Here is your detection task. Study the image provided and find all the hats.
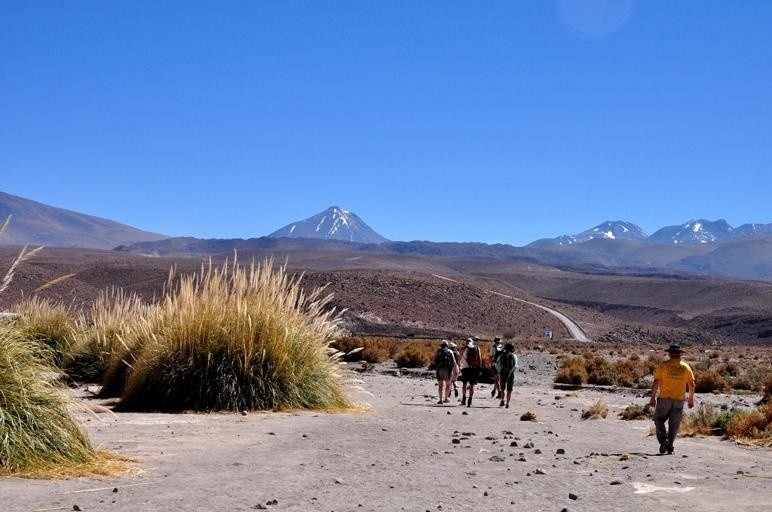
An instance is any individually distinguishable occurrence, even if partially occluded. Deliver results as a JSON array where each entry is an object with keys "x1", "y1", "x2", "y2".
[
  {"x1": 664, "y1": 343, "x2": 686, "y2": 355},
  {"x1": 440, "y1": 335, "x2": 501, "y2": 347}
]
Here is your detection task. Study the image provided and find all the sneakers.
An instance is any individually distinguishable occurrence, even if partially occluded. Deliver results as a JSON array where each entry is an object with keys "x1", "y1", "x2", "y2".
[
  {"x1": 659, "y1": 440, "x2": 675, "y2": 454},
  {"x1": 437, "y1": 388, "x2": 510, "y2": 409}
]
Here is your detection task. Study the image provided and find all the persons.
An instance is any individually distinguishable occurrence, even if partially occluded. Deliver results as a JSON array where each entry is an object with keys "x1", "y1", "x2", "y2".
[
  {"x1": 648, "y1": 344, "x2": 695, "y2": 453},
  {"x1": 495, "y1": 343, "x2": 518, "y2": 408},
  {"x1": 489, "y1": 337, "x2": 502, "y2": 398},
  {"x1": 457, "y1": 338, "x2": 482, "y2": 407},
  {"x1": 430, "y1": 340, "x2": 456, "y2": 404},
  {"x1": 448, "y1": 342, "x2": 460, "y2": 397}
]
